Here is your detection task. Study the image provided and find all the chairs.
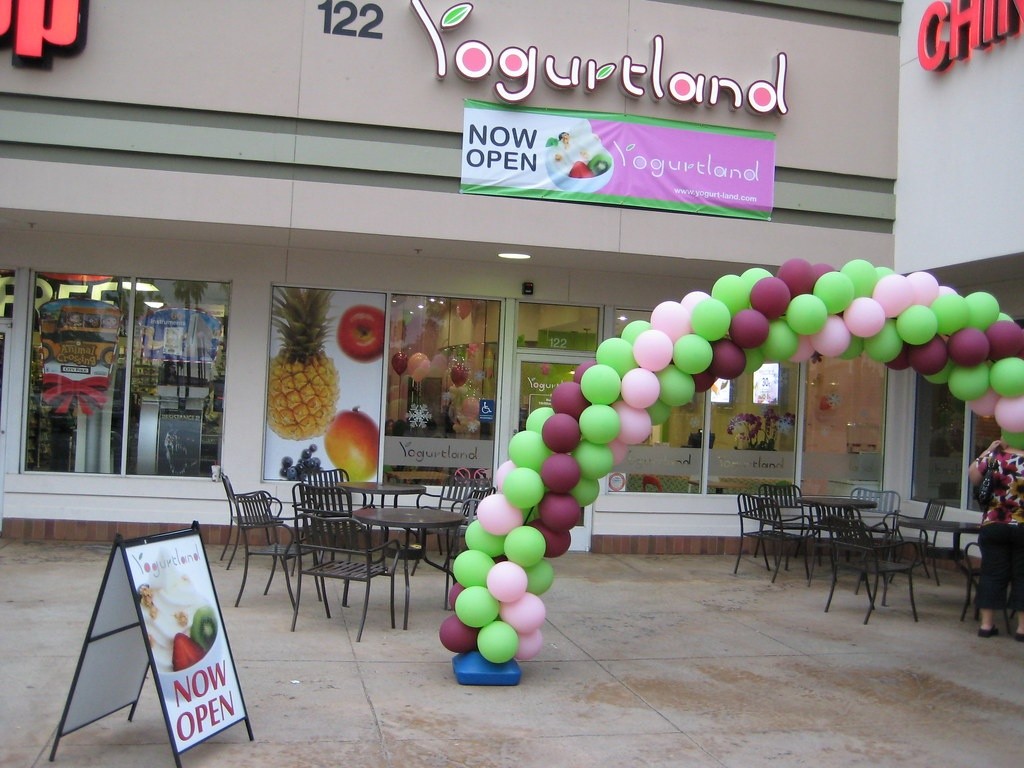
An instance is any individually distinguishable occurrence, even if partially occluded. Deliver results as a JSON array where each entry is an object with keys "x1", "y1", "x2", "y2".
[
  {"x1": 734, "y1": 484, "x2": 1015, "y2": 637},
  {"x1": 221, "y1": 468, "x2": 496, "y2": 641}
]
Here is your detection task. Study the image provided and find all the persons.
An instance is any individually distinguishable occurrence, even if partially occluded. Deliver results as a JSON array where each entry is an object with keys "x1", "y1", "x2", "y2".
[
  {"x1": 967, "y1": 435, "x2": 1024, "y2": 642},
  {"x1": 386, "y1": 417, "x2": 437, "y2": 435},
  {"x1": 519, "y1": 415, "x2": 528, "y2": 431}
]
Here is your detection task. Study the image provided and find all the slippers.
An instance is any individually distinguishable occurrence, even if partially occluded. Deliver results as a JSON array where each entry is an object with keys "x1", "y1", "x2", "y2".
[
  {"x1": 978, "y1": 624, "x2": 999, "y2": 637},
  {"x1": 1015, "y1": 633, "x2": 1024, "y2": 641}
]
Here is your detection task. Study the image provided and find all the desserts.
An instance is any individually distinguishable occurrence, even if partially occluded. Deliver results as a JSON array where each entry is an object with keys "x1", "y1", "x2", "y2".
[
  {"x1": 139, "y1": 546, "x2": 218, "y2": 673},
  {"x1": 548, "y1": 119, "x2": 601, "y2": 175}
]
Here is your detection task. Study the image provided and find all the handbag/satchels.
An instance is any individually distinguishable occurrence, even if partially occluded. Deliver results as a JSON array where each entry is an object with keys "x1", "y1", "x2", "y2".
[{"x1": 978, "y1": 447, "x2": 1000, "y2": 504}]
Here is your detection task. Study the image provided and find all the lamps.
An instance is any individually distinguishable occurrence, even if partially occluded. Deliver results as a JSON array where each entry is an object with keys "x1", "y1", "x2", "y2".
[{"x1": 568, "y1": 331, "x2": 577, "y2": 375}]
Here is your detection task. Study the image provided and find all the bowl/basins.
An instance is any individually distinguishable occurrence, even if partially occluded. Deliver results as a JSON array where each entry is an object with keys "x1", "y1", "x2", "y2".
[
  {"x1": 157, "y1": 625, "x2": 221, "y2": 701},
  {"x1": 546, "y1": 146, "x2": 613, "y2": 193}
]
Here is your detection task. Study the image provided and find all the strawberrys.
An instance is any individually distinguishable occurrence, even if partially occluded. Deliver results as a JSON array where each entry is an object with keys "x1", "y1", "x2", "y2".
[
  {"x1": 171, "y1": 633, "x2": 205, "y2": 672},
  {"x1": 568, "y1": 161, "x2": 595, "y2": 178}
]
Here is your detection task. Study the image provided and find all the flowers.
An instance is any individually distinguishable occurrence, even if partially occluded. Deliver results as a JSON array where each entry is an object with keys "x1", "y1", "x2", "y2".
[{"x1": 727, "y1": 407, "x2": 795, "y2": 450}]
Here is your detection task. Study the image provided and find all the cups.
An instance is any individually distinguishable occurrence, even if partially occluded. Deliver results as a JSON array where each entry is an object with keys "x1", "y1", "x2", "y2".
[{"x1": 211, "y1": 465, "x2": 221, "y2": 483}]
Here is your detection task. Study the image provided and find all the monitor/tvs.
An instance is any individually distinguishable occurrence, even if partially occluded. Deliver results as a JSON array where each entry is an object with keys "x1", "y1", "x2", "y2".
[
  {"x1": 710, "y1": 378, "x2": 732, "y2": 406},
  {"x1": 752, "y1": 362, "x2": 782, "y2": 407}
]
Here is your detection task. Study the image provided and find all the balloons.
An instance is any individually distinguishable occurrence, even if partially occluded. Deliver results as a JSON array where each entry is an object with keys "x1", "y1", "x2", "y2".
[
  {"x1": 819, "y1": 395, "x2": 831, "y2": 411},
  {"x1": 391, "y1": 352, "x2": 431, "y2": 382},
  {"x1": 450, "y1": 365, "x2": 468, "y2": 387},
  {"x1": 439, "y1": 257, "x2": 1024, "y2": 663},
  {"x1": 463, "y1": 397, "x2": 479, "y2": 420},
  {"x1": 457, "y1": 300, "x2": 472, "y2": 320}
]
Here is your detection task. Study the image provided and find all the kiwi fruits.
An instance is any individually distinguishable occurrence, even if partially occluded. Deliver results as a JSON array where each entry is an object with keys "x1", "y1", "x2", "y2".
[
  {"x1": 587, "y1": 153, "x2": 612, "y2": 176},
  {"x1": 189, "y1": 606, "x2": 217, "y2": 652}
]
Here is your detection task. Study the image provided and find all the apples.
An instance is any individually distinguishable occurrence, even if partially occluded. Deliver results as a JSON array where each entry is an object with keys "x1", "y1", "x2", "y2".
[
  {"x1": 337, "y1": 304, "x2": 384, "y2": 363},
  {"x1": 324, "y1": 407, "x2": 378, "y2": 482}
]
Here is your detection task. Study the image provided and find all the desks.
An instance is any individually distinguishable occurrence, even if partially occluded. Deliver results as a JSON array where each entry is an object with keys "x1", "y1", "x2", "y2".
[
  {"x1": 896, "y1": 518, "x2": 981, "y2": 621},
  {"x1": 795, "y1": 496, "x2": 878, "y2": 572},
  {"x1": 351, "y1": 508, "x2": 465, "y2": 630},
  {"x1": 336, "y1": 481, "x2": 426, "y2": 575},
  {"x1": 383, "y1": 468, "x2": 450, "y2": 485},
  {"x1": 689, "y1": 479, "x2": 747, "y2": 494}
]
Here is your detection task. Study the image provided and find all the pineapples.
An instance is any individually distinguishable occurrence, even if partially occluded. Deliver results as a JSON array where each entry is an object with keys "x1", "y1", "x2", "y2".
[{"x1": 267, "y1": 285, "x2": 342, "y2": 441}]
